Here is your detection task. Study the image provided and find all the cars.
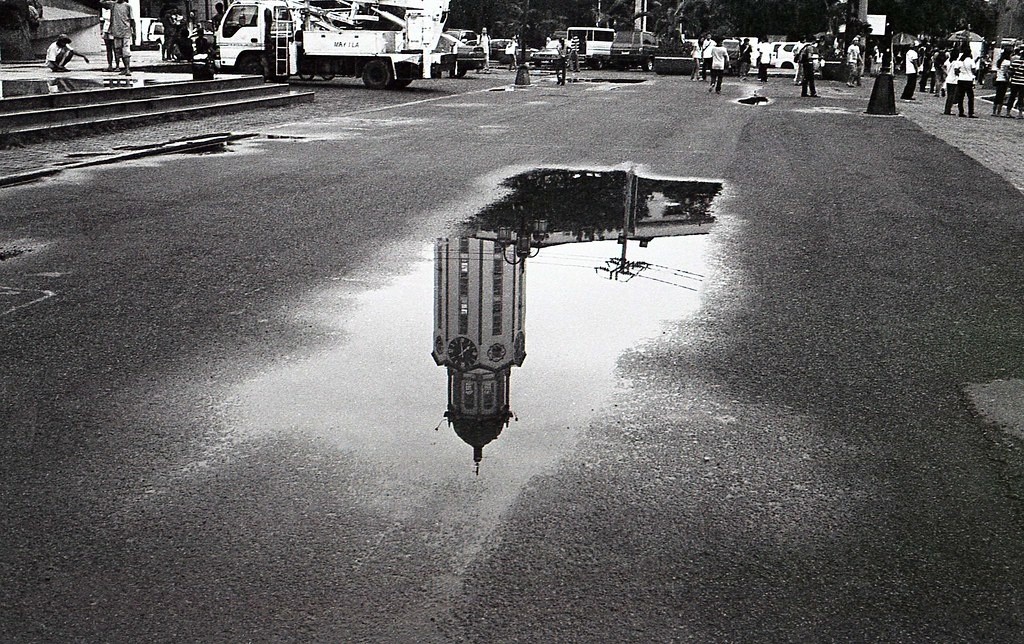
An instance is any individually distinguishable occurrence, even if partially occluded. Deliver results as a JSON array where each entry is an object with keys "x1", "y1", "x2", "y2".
[{"x1": 431, "y1": 27, "x2": 569, "y2": 78}]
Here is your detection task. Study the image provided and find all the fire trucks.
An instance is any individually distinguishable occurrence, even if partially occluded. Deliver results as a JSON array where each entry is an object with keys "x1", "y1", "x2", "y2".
[{"x1": 214, "y1": 0, "x2": 450, "y2": 91}]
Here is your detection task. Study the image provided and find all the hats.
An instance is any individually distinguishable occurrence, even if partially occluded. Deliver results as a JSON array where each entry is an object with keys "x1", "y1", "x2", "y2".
[{"x1": 57, "y1": 34, "x2": 72, "y2": 42}]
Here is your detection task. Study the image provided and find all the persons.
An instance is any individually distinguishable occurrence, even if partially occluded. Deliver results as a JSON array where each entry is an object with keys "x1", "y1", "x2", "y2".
[
  {"x1": 870, "y1": 40, "x2": 991, "y2": 118},
  {"x1": 991, "y1": 48, "x2": 1024, "y2": 119},
  {"x1": 555, "y1": 30, "x2": 580, "y2": 85},
  {"x1": 740, "y1": 36, "x2": 772, "y2": 82},
  {"x1": 505, "y1": 34, "x2": 518, "y2": 72},
  {"x1": 477, "y1": 28, "x2": 490, "y2": 74},
  {"x1": 29, "y1": 4, "x2": 40, "y2": 31},
  {"x1": 791, "y1": 35, "x2": 844, "y2": 97},
  {"x1": 690, "y1": 33, "x2": 730, "y2": 94},
  {"x1": 159, "y1": 0, "x2": 225, "y2": 60},
  {"x1": 546, "y1": 34, "x2": 552, "y2": 45},
  {"x1": 46, "y1": 34, "x2": 89, "y2": 71},
  {"x1": 846, "y1": 37, "x2": 866, "y2": 87},
  {"x1": 98, "y1": 0, "x2": 135, "y2": 76}
]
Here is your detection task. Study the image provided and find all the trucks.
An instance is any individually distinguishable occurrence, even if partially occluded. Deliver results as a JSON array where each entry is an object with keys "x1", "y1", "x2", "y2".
[
  {"x1": 139, "y1": 18, "x2": 166, "y2": 50},
  {"x1": 566, "y1": 26, "x2": 616, "y2": 70}
]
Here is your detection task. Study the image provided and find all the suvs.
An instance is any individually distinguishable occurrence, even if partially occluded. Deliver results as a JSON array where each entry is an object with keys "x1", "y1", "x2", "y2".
[
  {"x1": 689, "y1": 36, "x2": 800, "y2": 71},
  {"x1": 611, "y1": 31, "x2": 659, "y2": 73}
]
